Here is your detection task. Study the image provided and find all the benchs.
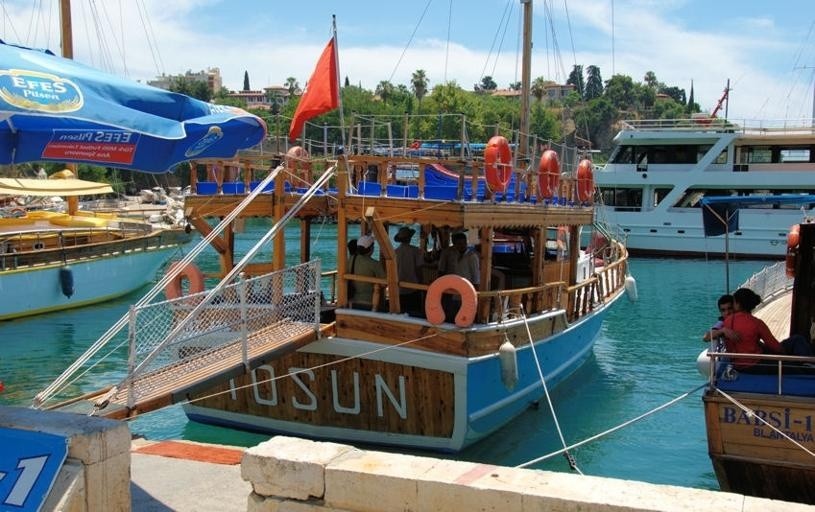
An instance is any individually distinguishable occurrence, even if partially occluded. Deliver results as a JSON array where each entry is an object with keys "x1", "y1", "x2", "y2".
[{"x1": 714, "y1": 352, "x2": 815, "y2": 397}]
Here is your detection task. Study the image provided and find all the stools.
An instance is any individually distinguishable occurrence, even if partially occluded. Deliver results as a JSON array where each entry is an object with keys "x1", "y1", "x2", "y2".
[{"x1": 494, "y1": 266, "x2": 513, "y2": 288}]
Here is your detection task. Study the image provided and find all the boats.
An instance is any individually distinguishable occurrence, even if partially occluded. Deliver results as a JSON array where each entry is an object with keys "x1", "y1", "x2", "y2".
[
  {"x1": 548, "y1": 107, "x2": 814, "y2": 262},
  {"x1": 695, "y1": 187, "x2": 815, "y2": 500}
]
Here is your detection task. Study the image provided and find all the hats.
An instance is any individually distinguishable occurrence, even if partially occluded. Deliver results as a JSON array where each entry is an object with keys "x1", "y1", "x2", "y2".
[
  {"x1": 357, "y1": 235, "x2": 374, "y2": 247},
  {"x1": 393, "y1": 226, "x2": 416, "y2": 242}
]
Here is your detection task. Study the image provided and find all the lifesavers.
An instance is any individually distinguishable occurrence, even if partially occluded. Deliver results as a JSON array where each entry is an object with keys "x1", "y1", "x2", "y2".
[
  {"x1": 287, "y1": 147, "x2": 314, "y2": 189},
  {"x1": 540, "y1": 150, "x2": 560, "y2": 199},
  {"x1": 164, "y1": 261, "x2": 203, "y2": 306},
  {"x1": 484, "y1": 136, "x2": 512, "y2": 194},
  {"x1": 577, "y1": 160, "x2": 594, "y2": 201},
  {"x1": 785, "y1": 224, "x2": 799, "y2": 279}
]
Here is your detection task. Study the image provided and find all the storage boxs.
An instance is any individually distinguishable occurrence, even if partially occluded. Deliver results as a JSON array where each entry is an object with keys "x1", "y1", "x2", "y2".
[{"x1": 194, "y1": 180, "x2": 418, "y2": 198}]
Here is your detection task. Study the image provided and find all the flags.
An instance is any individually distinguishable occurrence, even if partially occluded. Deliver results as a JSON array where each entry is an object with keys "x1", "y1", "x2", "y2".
[{"x1": 288, "y1": 36, "x2": 338, "y2": 142}]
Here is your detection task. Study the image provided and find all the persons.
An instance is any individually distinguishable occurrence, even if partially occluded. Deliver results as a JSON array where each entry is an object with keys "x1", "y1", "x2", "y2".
[
  {"x1": 347, "y1": 239, "x2": 356, "y2": 255},
  {"x1": 394, "y1": 227, "x2": 424, "y2": 317},
  {"x1": 444, "y1": 232, "x2": 480, "y2": 300},
  {"x1": 348, "y1": 236, "x2": 386, "y2": 311},
  {"x1": 723, "y1": 288, "x2": 815, "y2": 374},
  {"x1": 703, "y1": 295, "x2": 740, "y2": 342}
]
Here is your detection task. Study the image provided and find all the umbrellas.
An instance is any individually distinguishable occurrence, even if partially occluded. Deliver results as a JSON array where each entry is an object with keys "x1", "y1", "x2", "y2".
[{"x1": 1, "y1": 40, "x2": 267, "y2": 174}]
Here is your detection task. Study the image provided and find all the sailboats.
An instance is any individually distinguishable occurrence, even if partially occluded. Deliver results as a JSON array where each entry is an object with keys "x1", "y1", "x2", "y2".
[
  {"x1": 162, "y1": 0, "x2": 646, "y2": 451},
  {"x1": 0, "y1": 0, "x2": 203, "y2": 324}
]
